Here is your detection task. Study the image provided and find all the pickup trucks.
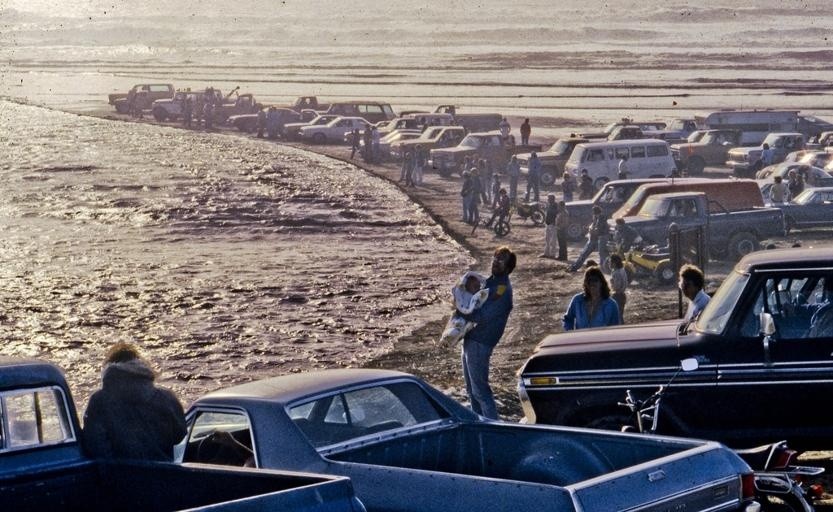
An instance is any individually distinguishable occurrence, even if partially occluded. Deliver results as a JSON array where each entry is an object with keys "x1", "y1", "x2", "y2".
[
  {"x1": 0, "y1": 357, "x2": 368, "y2": 512},
  {"x1": 107, "y1": 83, "x2": 543, "y2": 178},
  {"x1": 506, "y1": 111, "x2": 833, "y2": 199},
  {"x1": 172, "y1": 368, "x2": 758, "y2": 512},
  {"x1": 605, "y1": 192, "x2": 786, "y2": 259}
]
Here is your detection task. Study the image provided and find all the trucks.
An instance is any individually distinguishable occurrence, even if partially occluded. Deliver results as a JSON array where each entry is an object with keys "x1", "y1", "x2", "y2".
[{"x1": 587, "y1": 179, "x2": 765, "y2": 246}]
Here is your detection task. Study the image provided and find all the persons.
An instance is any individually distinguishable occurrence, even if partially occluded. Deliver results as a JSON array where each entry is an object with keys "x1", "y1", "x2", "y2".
[
  {"x1": 439, "y1": 273, "x2": 489, "y2": 347},
  {"x1": 561, "y1": 267, "x2": 622, "y2": 331},
  {"x1": 212, "y1": 430, "x2": 257, "y2": 468},
  {"x1": 449, "y1": 247, "x2": 515, "y2": 422},
  {"x1": 80, "y1": 340, "x2": 189, "y2": 463},
  {"x1": 134, "y1": 84, "x2": 833, "y2": 314},
  {"x1": 677, "y1": 265, "x2": 711, "y2": 318}
]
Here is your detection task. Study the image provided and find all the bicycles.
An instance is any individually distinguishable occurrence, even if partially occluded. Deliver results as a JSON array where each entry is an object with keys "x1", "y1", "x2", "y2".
[{"x1": 468, "y1": 208, "x2": 511, "y2": 237}]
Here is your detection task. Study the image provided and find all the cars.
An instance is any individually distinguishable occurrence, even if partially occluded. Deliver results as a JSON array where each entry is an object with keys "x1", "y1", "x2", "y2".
[{"x1": 765, "y1": 187, "x2": 833, "y2": 234}]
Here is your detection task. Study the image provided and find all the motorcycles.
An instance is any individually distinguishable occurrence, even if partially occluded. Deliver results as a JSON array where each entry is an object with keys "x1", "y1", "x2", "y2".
[
  {"x1": 616, "y1": 358, "x2": 825, "y2": 510},
  {"x1": 502, "y1": 198, "x2": 544, "y2": 226},
  {"x1": 602, "y1": 240, "x2": 656, "y2": 274},
  {"x1": 612, "y1": 248, "x2": 681, "y2": 284}
]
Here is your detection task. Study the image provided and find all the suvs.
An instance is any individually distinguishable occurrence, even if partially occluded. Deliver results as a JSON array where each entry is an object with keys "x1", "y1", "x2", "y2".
[
  {"x1": 519, "y1": 251, "x2": 832, "y2": 454},
  {"x1": 552, "y1": 180, "x2": 656, "y2": 237}
]
